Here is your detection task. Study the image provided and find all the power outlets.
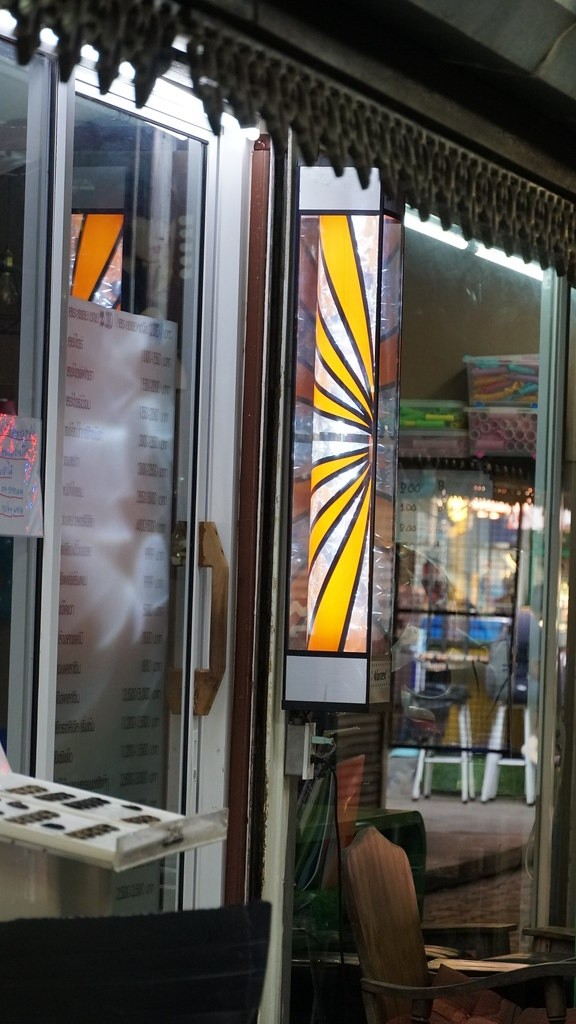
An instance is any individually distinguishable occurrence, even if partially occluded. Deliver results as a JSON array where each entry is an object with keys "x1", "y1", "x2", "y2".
[{"x1": 286, "y1": 724, "x2": 317, "y2": 781}]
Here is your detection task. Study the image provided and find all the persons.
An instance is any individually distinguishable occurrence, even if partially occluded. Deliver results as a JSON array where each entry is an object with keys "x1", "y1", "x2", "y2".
[
  {"x1": 394, "y1": 559, "x2": 459, "y2": 742},
  {"x1": 485, "y1": 582, "x2": 543, "y2": 706}
]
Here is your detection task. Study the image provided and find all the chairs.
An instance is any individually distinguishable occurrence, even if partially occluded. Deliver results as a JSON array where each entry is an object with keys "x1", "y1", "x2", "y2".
[
  {"x1": 342, "y1": 827, "x2": 576, "y2": 1024},
  {"x1": 355, "y1": 811, "x2": 517, "y2": 968},
  {"x1": 0, "y1": 899, "x2": 272, "y2": 1024}
]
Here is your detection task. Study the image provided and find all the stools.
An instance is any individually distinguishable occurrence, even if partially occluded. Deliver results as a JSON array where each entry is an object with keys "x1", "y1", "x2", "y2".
[
  {"x1": 410, "y1": 686, "x2": 475, "y2": 803},
  {"x1": 476, "y1": 695, "x2": 536, "y2": 803}
]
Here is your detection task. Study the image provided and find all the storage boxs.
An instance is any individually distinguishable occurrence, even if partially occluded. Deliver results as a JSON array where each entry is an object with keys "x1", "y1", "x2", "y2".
[
  {"x1": 399, "y1": 400, "x2": 538, "y2": 461},
  {"x1": 462, "y1": 352, "x2": 540, "y2": 408}
]
